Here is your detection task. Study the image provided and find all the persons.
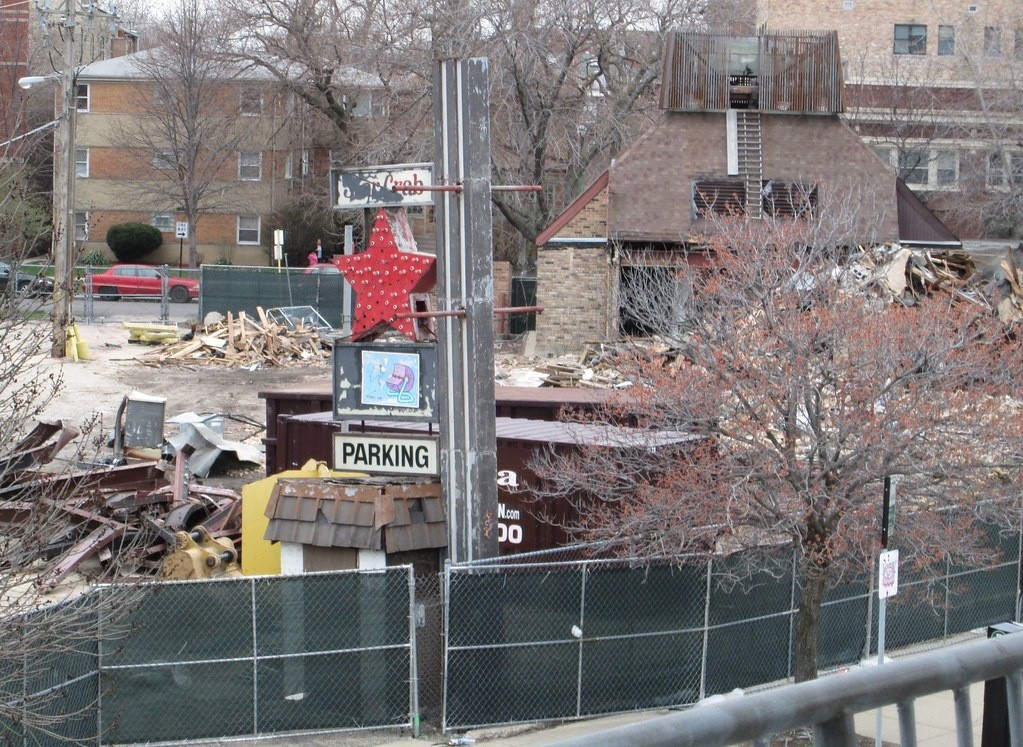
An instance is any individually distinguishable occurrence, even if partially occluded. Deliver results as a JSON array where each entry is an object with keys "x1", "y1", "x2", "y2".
[{"x1": 313, "y1": 238, "x2": 325, "y2": 263}]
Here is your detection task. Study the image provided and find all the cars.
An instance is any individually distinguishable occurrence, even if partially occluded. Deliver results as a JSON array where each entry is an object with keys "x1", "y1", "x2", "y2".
[
  {"x1": 81, "y1": 263, "x2": 201, "y2": 304},
  {"x1": 0, "y1": 260, "x2": 55, "y2": 300}
]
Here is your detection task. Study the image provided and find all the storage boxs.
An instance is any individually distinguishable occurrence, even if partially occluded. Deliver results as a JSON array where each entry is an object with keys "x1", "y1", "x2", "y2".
[{"x1": 259, "y1": 384, "x2": 720, "y2": 557}]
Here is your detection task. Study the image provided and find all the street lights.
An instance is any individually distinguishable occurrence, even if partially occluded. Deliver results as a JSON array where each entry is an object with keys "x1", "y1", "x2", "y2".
[{"x1": 16, "y1": 74, "x2": 74, "y2": 358}]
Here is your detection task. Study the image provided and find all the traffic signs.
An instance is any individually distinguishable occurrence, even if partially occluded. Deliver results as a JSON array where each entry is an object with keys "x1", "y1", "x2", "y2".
[{"x1": 175, "y1": 221, "x2": 188, "y2": 239}]
